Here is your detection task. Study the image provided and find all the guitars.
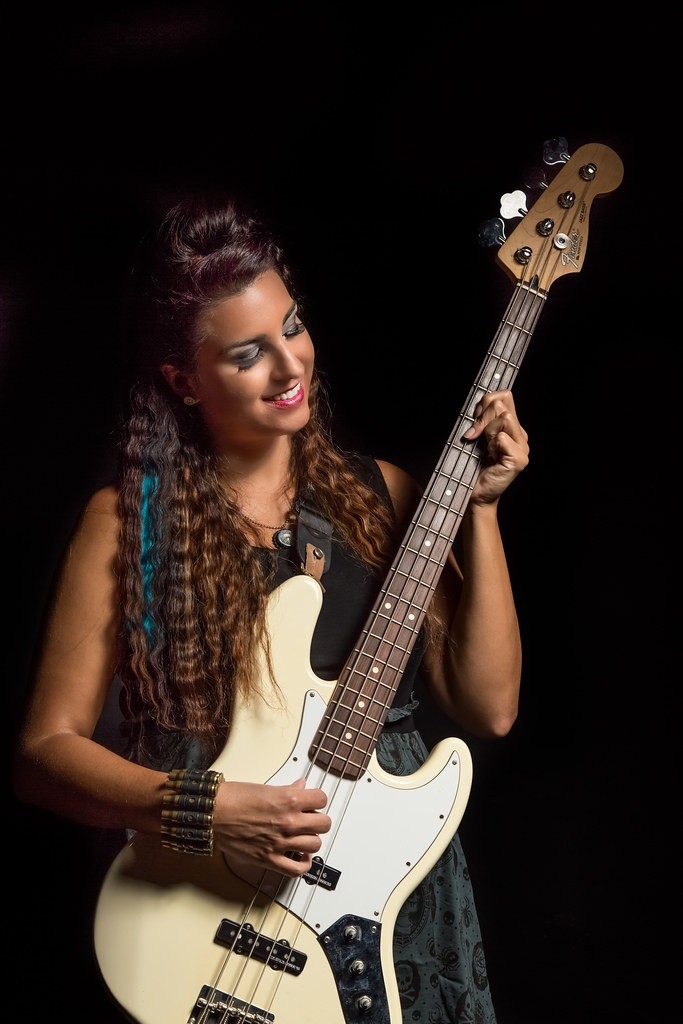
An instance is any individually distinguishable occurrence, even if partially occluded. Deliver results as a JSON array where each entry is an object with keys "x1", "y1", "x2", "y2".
[{"x1": 91, "y1": 141, "x2": 627, "y2": 1024}]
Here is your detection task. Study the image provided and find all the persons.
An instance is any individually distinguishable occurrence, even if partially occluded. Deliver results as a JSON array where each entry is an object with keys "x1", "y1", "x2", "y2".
[{"x1": 13, "y1": 190, "x2": 531, "y2": 1024}]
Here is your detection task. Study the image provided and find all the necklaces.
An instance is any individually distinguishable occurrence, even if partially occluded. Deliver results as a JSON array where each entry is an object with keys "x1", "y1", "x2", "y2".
[{"x1": 218, "y1": 490, "x2": 298, "y2": 549}]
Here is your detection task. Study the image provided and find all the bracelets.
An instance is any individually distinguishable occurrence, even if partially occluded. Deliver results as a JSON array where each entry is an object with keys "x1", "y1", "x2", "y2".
[{"x1": 160, "y1": 768, "x2": 225, "y2": 857}]
